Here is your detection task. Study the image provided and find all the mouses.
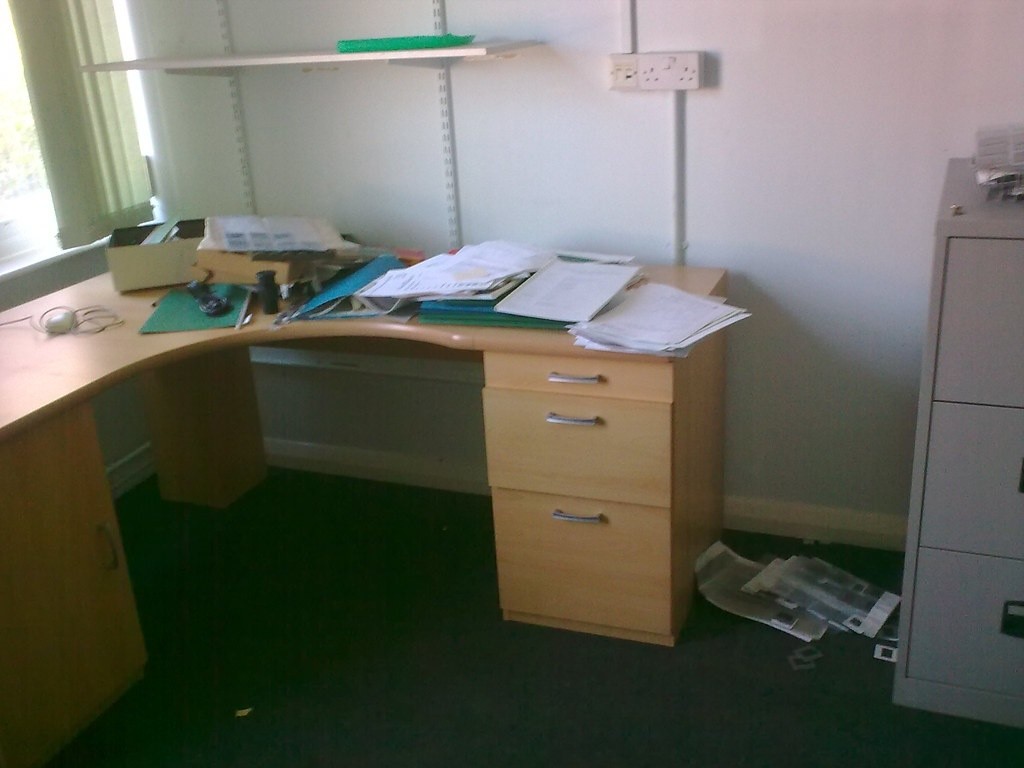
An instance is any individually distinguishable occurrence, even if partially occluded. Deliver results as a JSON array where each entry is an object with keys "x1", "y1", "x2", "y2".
[{"x1": 45, "y1": 312, "x2": 76, "y2": 334}]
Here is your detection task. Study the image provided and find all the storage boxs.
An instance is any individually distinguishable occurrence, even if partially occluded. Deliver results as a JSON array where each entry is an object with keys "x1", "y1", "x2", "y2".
[
  {"x1": 198, "y1": 248, "x2": 334, "y2": 286},
  {"x1": 105, "y1": 218, "x2": 206, "y2": 292}
]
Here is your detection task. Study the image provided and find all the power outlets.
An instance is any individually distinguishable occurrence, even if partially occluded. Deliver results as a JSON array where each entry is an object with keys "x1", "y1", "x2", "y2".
[{"x1": 610, "y1": 51, "x2": 707, "y2": 91}]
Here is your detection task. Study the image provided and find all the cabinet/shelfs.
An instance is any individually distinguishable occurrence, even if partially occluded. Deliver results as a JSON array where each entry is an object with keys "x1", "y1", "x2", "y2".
[
  {"x1": 892, "y1": 159, "x2": 1024, "y2": 727},
  {"x1": 0, "y1": 259, "x2": 728, "y2": 768}
]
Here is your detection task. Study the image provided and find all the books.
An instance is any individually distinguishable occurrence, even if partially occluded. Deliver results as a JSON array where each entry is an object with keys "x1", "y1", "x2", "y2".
[{"x1": 198, "y1": 209, "x2": 753, "y2": 359}]
